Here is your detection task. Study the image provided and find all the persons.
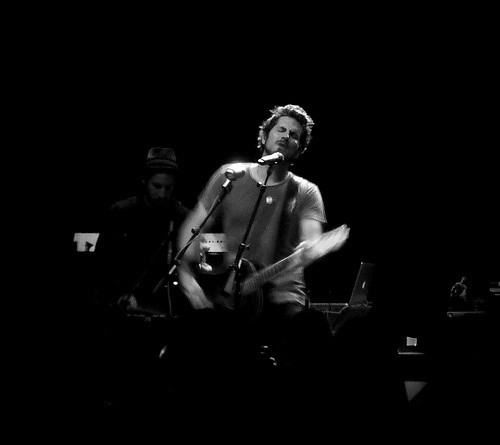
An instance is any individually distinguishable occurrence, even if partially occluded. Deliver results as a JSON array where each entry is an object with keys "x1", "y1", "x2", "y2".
[
  {"x1": 176, "y1": 103, "x2": 328, "y2": 405},
  {"x1": 91, "y1": 146, "x2": 189, "y2": 299}
]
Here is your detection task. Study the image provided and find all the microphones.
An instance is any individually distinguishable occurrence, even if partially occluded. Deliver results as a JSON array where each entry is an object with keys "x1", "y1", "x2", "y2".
[{"x1": 258, "y1": 152, "x2": 285, "y2": 165}]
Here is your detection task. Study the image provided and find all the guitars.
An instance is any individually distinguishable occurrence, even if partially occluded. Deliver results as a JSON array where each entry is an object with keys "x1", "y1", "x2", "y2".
[{"x1": 167, "y1": 221, "x2": 350, "y2": 344}]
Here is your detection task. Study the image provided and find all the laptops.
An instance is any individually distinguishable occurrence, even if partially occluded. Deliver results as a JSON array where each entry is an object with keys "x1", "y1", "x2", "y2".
[{"x1": 310, "y1": 262, "x2": 374, "y2": 306}]
